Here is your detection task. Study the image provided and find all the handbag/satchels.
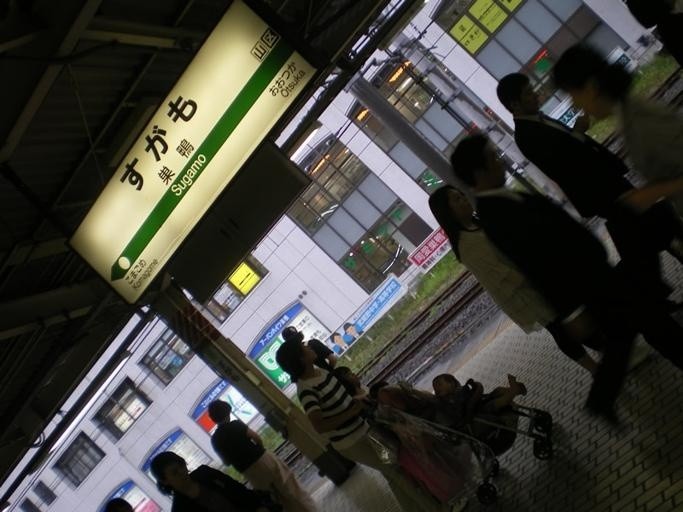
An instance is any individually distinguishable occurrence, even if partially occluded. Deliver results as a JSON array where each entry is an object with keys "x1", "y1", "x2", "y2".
[
  {"x1": 364, "y1": 422, "x2": 402, "y2": 466},
  {"x1": 399, "y1": 435, "x2": 467, "y2": 506}
]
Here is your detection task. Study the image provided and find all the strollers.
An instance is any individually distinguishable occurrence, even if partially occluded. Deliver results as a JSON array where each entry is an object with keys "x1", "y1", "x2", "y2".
[{"x1": 365, "y1": 380, "x2": 556, "y2": 504}]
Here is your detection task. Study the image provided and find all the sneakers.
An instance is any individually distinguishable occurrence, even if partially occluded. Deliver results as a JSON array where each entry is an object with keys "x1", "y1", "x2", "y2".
[
  {"x1": 506, "y1": 370, "x2": 529, "y2": 397},
  {"x1": 572, "y1": 342, "x2": 656, "y2": 437}
]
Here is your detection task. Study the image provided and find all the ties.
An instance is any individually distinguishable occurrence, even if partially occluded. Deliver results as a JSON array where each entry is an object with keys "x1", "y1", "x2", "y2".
[{"x1": 504, "y1": 187, "x2": 533, "y2": 203}]
